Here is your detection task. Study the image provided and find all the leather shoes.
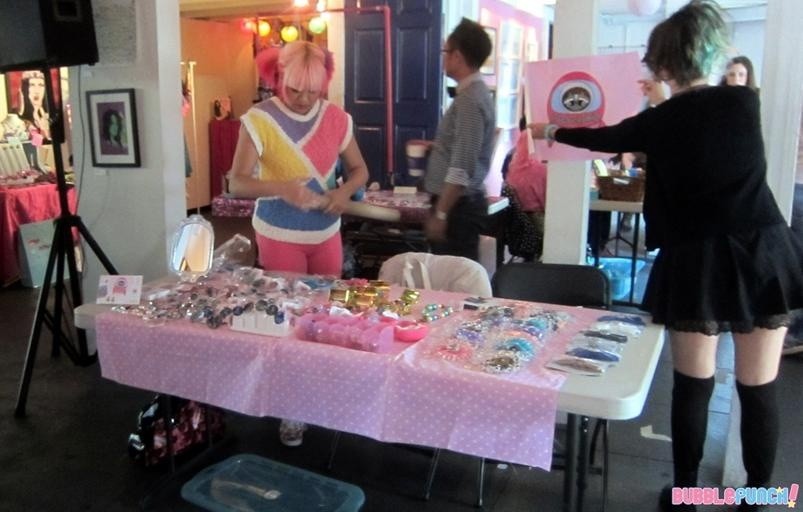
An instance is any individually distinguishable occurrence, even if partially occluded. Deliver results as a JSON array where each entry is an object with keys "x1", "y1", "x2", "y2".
[{"x1": 660, "y1": 483, "x2": 694, "y2": 511}]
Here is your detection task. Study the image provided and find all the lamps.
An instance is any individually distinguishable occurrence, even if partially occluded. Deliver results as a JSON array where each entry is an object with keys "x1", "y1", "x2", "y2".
[{"x1": 0, "y1": 0, "x2": 120, "y2": 419}]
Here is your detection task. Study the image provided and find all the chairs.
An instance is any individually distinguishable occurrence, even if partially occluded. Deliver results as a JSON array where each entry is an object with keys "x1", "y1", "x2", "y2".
[
  {"x1": 479, "y1": 262, "x2": 610, "y2": 511},
  {"x1": 327, "y1": 252, "x2": 493, "y2": 500}
]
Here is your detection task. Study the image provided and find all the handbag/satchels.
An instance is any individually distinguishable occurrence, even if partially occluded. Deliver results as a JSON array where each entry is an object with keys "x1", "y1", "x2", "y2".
[{"x1": 125, "y1": 392, "x2": 226, "y2": 470}]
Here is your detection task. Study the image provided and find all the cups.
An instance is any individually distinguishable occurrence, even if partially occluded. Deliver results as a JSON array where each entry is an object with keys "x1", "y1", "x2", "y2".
[{"x1": 406, "y1": 144, "x2": 427, "y2": 178}]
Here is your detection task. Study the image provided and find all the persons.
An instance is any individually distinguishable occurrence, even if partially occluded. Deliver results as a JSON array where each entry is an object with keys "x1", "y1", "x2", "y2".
[
  {"x1": 100, "y1": 109, "x2": 121, "y2": 147},
  {"x1": 419, "y1": 15, "x2": 496, "y2": 262},
  {"x1": 718, "y1": 54, "x2": 755, "y2": 89},
  {"x1": 499, "y1": 115, "x2": 526, "y2": 180},
  {"x1": 503, "y1": 129, "x2": 548, "y2": 233},
  {"x1": 227, "y1": 39, "x2": 372, "y2": 447},
  {"x1": 607, "y1": 151, "x2": 646, "y2": 234},
  {"x1": 18, "y1": 69, "x2": 59, "y2": 146},
  {"x1": 523, "y1": 0, "x2": 802, "y2": 511}
]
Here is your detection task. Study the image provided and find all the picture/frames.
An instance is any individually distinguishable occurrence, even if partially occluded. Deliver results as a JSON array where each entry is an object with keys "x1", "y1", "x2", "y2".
[
  {"x1": 480, "y1": 21, "x2": 523, "y2": 131},
  {"x1": 85, "y1": 88, "x2": 141, "y2": 168}
]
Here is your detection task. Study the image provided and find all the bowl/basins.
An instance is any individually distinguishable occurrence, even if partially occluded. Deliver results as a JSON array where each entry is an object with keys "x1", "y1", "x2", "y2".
[{"x1": 395, "y1": 321, "x2": 430, "y2": 341}]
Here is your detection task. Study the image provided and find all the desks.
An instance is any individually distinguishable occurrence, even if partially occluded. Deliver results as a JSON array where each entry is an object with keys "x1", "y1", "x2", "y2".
[
  {"x1": 496, "y1": 176, "x2": 646, "y2": 306},
  {"x1": 73, "y1": 270, "x2": 665, "y2": 512},
  {"x1": 211, "y1": 190, "x2": 510, "y2": 277}
]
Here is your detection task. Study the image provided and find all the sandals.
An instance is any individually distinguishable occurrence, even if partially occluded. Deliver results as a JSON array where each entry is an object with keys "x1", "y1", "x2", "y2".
[{"x1": 279, "y1": 418, "x2": 305, "y2": 448}]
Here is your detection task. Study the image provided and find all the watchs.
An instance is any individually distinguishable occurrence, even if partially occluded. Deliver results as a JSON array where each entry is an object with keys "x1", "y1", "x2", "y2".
[{"x1": 431, "y1": 210, "x2": 450, "y2": 222}]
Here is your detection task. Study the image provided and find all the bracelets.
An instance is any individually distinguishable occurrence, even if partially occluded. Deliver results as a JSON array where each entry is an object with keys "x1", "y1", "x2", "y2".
[
  {"x1": 416, "y1": 300, "x2": 454, "y2": 327},
  {"x1": 544, "y1": 122, "x2": 558, "y2": 143}
]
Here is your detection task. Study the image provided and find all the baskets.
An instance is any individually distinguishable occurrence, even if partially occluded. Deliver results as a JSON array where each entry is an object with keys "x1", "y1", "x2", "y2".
[{"x1": 596, "y1": 170, "x2": 646, "y2": 201}]
